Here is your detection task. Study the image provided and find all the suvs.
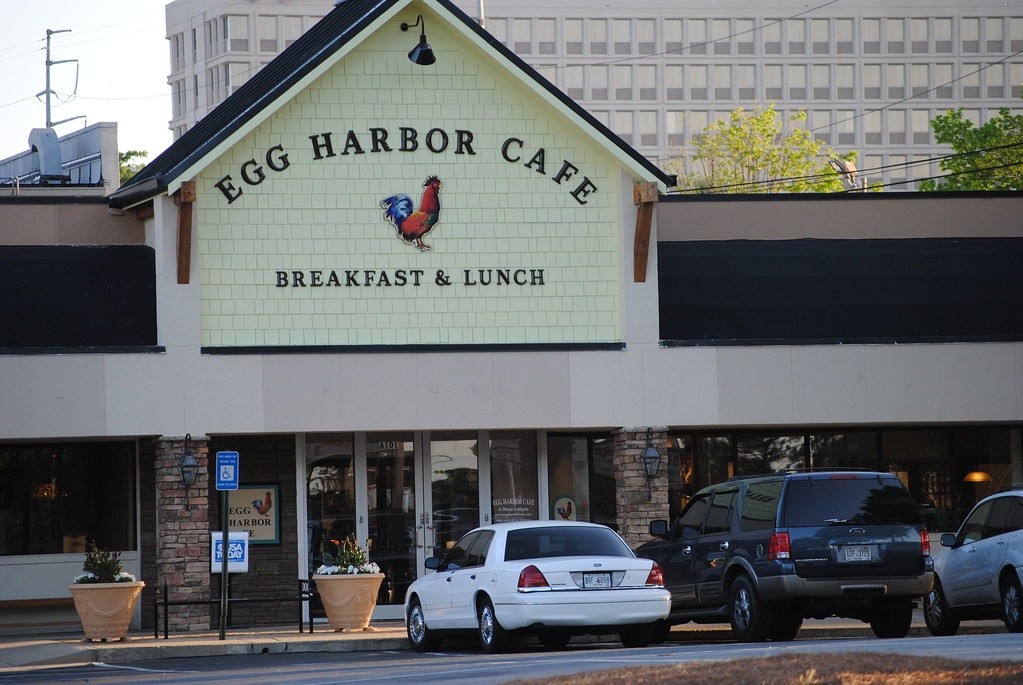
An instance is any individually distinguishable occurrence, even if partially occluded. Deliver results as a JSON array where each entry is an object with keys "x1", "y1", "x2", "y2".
[{"x1": 619, "y1": 466, "x2": 938, "y2": 648}]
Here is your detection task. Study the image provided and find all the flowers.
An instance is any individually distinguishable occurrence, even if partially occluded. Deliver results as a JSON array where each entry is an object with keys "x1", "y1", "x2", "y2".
[
  {"x1": 313, "y1": 531, "x2": 380, "y2": 574},
  {"x1": 73, "y1": 535, "x2": 136, "y2": 584}
]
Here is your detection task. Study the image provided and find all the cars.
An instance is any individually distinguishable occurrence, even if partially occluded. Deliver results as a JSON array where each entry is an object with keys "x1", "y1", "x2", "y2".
[
  {"x1": 403, "y1": 521, "x2": 672, "y2": 654},
  {"x1": 923, "y1": 488, "x2": 1023, "y2": 637}
]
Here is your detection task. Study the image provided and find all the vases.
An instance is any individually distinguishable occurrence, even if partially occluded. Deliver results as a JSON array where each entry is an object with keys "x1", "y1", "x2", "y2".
[
  {"x1": 68, "y1": 581, "x2": 144, "y2": 643},
  {"x1": 311, "y1": 573, "x2": 387, "y2": 633}
]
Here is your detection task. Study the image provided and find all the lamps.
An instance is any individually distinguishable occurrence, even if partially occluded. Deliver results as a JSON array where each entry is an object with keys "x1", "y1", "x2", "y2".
[
  {"x1": 962, "y1": 458, "x2": 994, "y2": 482},
  {"x1": 178, "y1": 432, "x2": 199, "y2": 512},
  {"x1": 400, "y1": 14, "x2": 437, "y2": 66},
  {"x1": 639, "y1": 428, "x2": 663, "y2": 502}
]
